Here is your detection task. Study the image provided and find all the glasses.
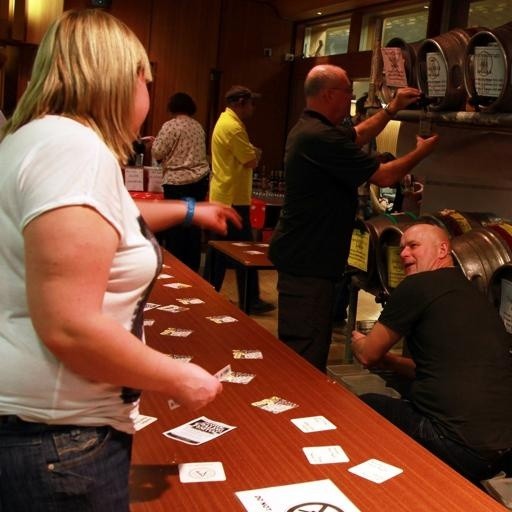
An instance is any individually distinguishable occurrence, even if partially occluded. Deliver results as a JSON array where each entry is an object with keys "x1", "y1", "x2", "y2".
[{"x1": 329, "y1": 86, "x2": 353, "y2": 93}]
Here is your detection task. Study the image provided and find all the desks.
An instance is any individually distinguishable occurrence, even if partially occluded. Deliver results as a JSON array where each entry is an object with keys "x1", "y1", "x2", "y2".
[
  {"x1": 127, "y1": 246, "x2": 512, "y2": 512},
  {"x1": 249, "y1": 199, "x2": 287, "y2": 229},
  {"x1": 128, "y1": 192, "x2": 165, "y2": 201},
  {"x1": 208, "y1": 240, "x2": 276, "y2": 316}
]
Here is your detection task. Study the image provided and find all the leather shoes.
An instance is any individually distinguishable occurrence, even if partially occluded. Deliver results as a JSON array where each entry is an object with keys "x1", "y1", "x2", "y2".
[{"x1": 238, "y1": 297, "x2": 276, "y2": 315}]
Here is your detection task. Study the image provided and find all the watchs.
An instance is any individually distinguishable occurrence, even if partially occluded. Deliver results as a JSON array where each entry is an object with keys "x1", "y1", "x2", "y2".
[
  {"x1": 179, "y1": 197, "x2": 196, "y2": 226},
  {"x1": 384, "y1": 103, "x2": 393, "y2": 117}
]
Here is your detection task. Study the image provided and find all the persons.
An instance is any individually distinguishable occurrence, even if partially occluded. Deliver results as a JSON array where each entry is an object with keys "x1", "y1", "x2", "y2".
[
  {"x1": 203, "y1": 85, "x2": 275, "y2": 314},
  {"x1": 266, "y1": 64, "x2": 441, "y2": 371},
  {"x1": 352, "y1": 223, "x2": 511, "y2": 483},
  {"x1": 140, "y1": 92, "x2": 210, "y2": 270},
  {"x1": 0, "y1": 10, "x2": 222, "y2": 512}
]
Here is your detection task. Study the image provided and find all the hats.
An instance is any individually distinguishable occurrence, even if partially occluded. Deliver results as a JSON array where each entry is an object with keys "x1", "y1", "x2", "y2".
[{"x1": 224, "y1": 85, "x2": 262, "y2": 104}]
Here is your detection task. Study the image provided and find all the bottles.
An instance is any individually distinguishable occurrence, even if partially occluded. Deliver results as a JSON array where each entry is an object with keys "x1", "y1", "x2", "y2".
[
  {"x1": 135, "y1": 140, "x2": 144, "y2": 167},
  {"x1": 252, "y1": 165, "x2": 285, "y2": 200}
]
